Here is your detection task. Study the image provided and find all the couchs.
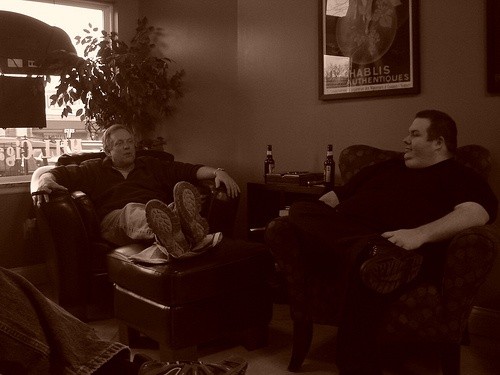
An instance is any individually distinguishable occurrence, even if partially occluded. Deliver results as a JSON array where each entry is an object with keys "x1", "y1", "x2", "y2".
[
  {"x1": 264, "y1": 145, "x2": 500, "y2": 375},
  {"x1": 33, "y1": 147, "x2": 242, "y2": 323}
]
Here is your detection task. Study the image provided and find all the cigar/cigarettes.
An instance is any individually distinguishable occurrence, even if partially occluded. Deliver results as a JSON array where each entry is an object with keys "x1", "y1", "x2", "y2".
[{"x1": 33, "y1": 190, "x2": 50, "y2": 194}]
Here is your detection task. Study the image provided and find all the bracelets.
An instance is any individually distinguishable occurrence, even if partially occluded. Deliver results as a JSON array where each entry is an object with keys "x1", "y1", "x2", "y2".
[{"x1": 213, "y1": 168, "x2": 224, "y2": 175}]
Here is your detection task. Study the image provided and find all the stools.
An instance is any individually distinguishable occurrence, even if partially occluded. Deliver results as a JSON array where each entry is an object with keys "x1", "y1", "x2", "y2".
[{"x1": 105, "y1": 231, "x2": 269, "y2": 364}]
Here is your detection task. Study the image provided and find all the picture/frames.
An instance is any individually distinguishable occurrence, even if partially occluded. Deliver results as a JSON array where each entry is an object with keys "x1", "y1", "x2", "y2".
[{"x1": 316, "y1": 0, "x2": 421, "y2": 101}]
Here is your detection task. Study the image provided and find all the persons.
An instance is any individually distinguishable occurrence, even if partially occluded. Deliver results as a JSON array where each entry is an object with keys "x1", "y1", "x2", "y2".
[
  {"x1": 38, "y1": 123, "x2": 241, "y2": 260},
  {"x1": 287, "y1": 109, "x2": 498, "y2": 375}
]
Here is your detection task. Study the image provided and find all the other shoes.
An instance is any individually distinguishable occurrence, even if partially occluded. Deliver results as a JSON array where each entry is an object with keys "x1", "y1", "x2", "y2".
[
  {"x1": 133, "y1": 353, "x2": 248, "y2": 375},
  {"x1": 172, "y1": 181, "x2": 209, "y2": 244},
  {"x1": 144, "y1": 199, "x2": 187, "y2": 259},
  {"x1": 360, "y1": 235, "x2": 423, "y2": 294}
]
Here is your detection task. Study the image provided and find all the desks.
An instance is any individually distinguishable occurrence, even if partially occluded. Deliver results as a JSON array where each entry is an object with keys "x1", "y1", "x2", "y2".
[{"x1": 236, "y1": 171, "x2": 346, "y2": 303}]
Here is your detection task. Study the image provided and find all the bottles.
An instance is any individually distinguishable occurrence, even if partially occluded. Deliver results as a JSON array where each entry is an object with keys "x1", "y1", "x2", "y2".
[
  {"x1": 263, "y1": 145, "x2": 276, "y2": 182},
  {"x1": 323, "y1": 144, "x2": 335, "y2": 186}
]
